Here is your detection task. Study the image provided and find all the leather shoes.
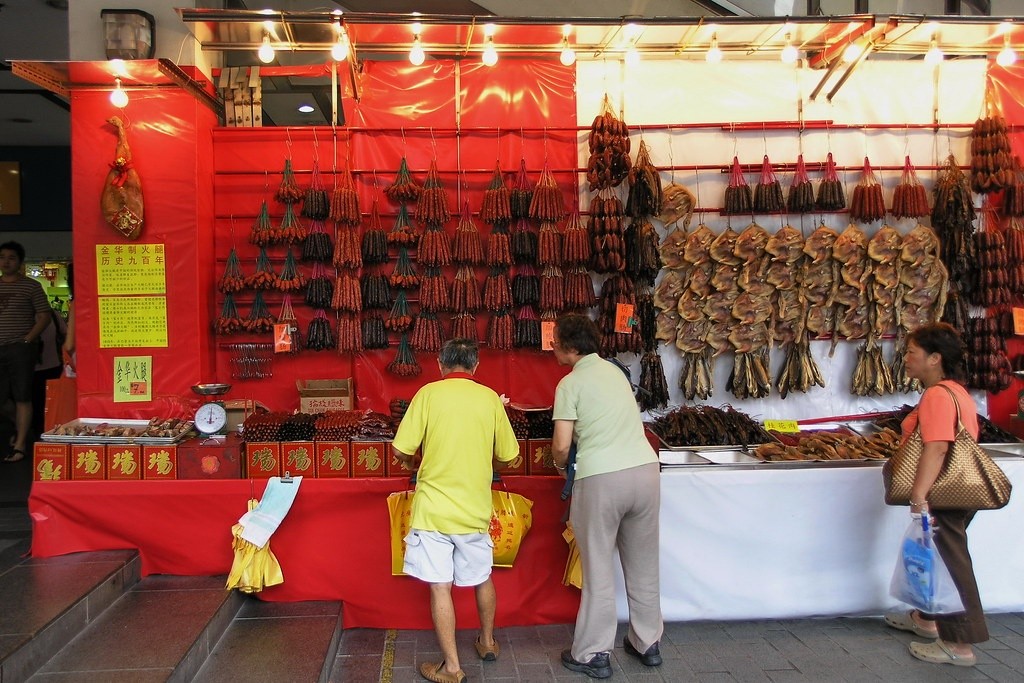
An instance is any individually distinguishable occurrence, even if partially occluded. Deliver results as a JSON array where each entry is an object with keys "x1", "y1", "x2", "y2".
[
  {"x1": 419, "y1": 659, "x2": 468, "y2": 683},
  {"x1": 474, "y1": 633, "x2": 500, "y2": 662}
]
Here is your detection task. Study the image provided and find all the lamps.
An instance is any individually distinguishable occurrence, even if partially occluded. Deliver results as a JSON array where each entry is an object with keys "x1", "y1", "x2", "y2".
[{"x1": 100, "y1": 9, "x2": 156, "y2": 60}]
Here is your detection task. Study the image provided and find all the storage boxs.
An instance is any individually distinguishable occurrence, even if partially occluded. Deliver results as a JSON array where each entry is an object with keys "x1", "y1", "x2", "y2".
[
  {"x1": 34, "y1": 433, "x2": 198, "y2": 481},
  {"x1": 296, "y1": 377, "x2": 354, "y2": 415},
  {"x1": 245, "y1": 438, "x2": 558, "y2": 479},
  {"x1": 226, "y1": 408, "x2": 253, "y2": 431}
]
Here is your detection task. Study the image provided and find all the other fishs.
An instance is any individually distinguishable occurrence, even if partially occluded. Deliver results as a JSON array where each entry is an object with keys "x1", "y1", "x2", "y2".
[{"x1": 680, "y1": 345, "x2": 925, "y2": 400}]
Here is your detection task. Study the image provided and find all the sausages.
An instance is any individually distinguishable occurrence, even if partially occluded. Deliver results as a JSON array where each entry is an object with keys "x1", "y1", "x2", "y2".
[
  {"x1": 212, "y1": 184, "x2": 307, "y2": 335},
  {"x1": 504, "y1": 404, "x2": 552, "y2": 438},
  {"x1": 724, "y1": 180, "x2": 846, "y2": 214},
  {"x1": 972, "y1": 115, "x2": 1024, "y2": 393},
  {"x1": 851, "y1": 184, "x2": 930, "y2": 226},
  {"x1": 241, "y1": 399, "x2": 408, "y2": 444},
  {"x1": 586, "y1": 114, "x2": 642, "y2": 359},
  {"x1": 300, "y1": 188, "x2": 392, "y2": 352},
  {"x1": 413, "y1": 185, "x2": 596, "y2": 354},
  {"x1": 382, "y1": 181, "x2": 423, "y2": 377}
]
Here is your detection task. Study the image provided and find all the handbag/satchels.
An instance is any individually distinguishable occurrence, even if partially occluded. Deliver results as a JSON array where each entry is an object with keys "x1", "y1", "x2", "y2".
[
  {"x1": 881, "y1": 384, "x2": 1013, "y2": 510},
  {"x1": 386, "y1": 473, "x2": 534, "y2": 576},
  {"x1": 888, "y1": 514, "x2": 965, "y2": 616}
]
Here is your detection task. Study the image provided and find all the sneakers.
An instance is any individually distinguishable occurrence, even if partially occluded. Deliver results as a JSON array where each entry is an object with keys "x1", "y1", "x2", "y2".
[
  {"x1": 561, "y1": 649, "x2": 613, "y2": 678},
  {"x1": 623, "y1": 634, "x2": 663, "y2": 666}
]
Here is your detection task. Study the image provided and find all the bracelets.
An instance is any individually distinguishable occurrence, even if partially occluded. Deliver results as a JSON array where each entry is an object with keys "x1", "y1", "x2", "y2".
[
  {"x1": 552, "y1": 460, "x2": 567, "y2": 470},
  {"x1": 24, "y1": 337, "x2": 32, "y2": 343},
  {"x1": 909, "y1": 500, "x2": 928, "y2": 507}
]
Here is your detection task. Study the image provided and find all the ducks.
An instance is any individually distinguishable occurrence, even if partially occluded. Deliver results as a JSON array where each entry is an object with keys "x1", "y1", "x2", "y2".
[{"x1": 655, "y1": 184, "x2": 948, "y2": 357}]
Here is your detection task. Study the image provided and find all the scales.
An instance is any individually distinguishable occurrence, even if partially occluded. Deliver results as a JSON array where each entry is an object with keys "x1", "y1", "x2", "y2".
[{"x1": 192, "y1": 382, "x2": 231, "y2": 439}]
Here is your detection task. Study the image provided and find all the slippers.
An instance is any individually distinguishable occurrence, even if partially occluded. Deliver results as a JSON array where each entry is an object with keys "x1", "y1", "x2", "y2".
[
  {"x1": 3, "y1": 449, "x2": 30, "y2": 464},
  {"x1": 883, "y1": 609, "x2": 939, "y2": 639},
  {"x1": 909, "y1": 638, "x2": 976, "y2": 666}
]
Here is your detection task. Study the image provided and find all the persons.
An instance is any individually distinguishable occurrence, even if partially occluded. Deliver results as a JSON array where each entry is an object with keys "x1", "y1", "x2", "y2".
[
  {"x1": 883, "y1": 320, "x2": 991, "y2": 666},
  {"x1": 0, "y1": 241, "x2": 74, "y2": 463},
  {"x1": 390, "y1": 336, "x2": 521, "y2": 683},
  {"x1": 551, "y1": 313, "x2": 664, "y2": 679}
]
